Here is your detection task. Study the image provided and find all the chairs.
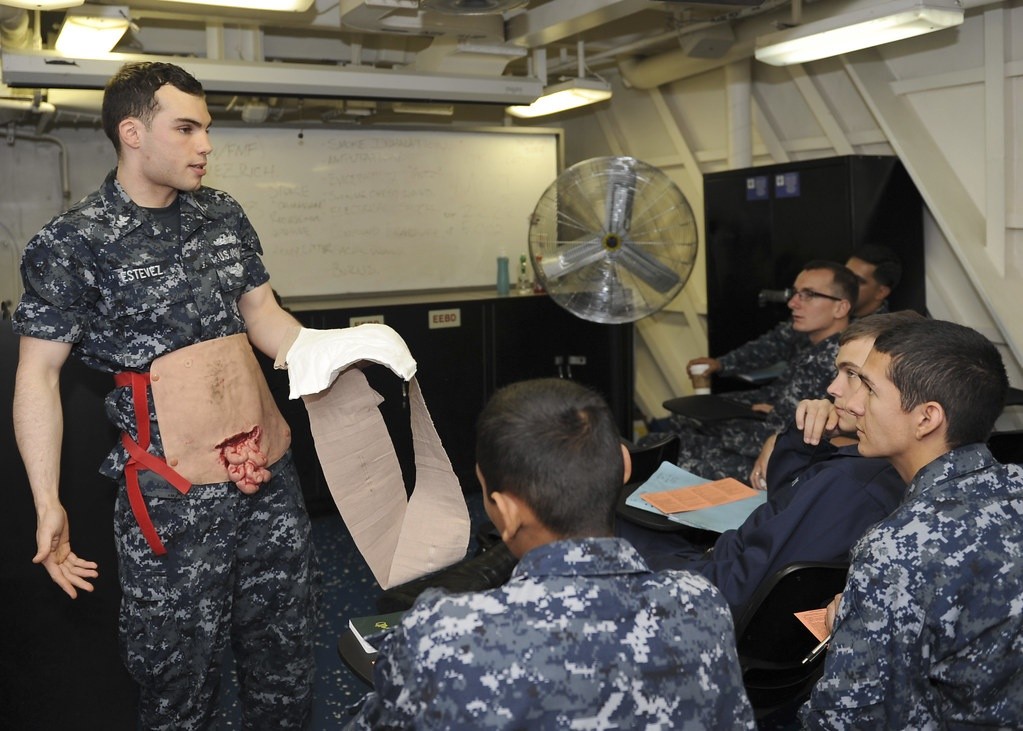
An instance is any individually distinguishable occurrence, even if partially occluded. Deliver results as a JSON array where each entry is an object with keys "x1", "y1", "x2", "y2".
[{"x1": 736, "y1": 560, "x2": 849, "y2": 731}]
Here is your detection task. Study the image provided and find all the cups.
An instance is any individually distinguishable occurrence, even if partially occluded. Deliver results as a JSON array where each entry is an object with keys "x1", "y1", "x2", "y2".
[
  {"x1": 690, "y1": 363, "x2": 711, "y2": 395},
  {"x1": 497, "y1": 256, "x2": 509, "y2": 295}
]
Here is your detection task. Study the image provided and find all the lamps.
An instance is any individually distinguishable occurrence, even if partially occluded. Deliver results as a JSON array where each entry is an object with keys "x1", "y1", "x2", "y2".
[
  {"x1": 54, "y1": 3, "x2": 131, "y2": 57},
  {"x1": 504, "y1": 36, "x2": 613, "y2": 119},
  {"x1": 753, "y1": 0, "x2": 967, "y2": 67}
]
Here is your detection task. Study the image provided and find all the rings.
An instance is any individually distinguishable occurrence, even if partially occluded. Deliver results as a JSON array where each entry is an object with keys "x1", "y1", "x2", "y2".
[{"x1": 754, "y1": 472, "x2": 760, "y2": 475}]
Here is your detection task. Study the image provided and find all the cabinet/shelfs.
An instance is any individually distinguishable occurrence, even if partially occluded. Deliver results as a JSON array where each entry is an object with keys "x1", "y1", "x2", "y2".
[{"x1": 247, "y1": 284, "x2": 635, "y2": 521}]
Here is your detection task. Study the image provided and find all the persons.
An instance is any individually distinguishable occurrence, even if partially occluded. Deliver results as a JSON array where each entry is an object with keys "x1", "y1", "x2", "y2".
[
  {"x1": 639, "y1": 310, "x2": 1023, "y2": 731},
  {"x1": 12, "y1": 61, "x2": 312, "y2": 731},
  {"x1": 341, "y1": 377, "x2": 759, "y2": 731},
  {"x1": 636, "y1": 236, "x2": 902, "y2": 490}
]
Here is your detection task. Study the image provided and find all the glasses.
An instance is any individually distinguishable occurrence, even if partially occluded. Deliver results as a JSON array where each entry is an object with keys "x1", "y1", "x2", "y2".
[
  {"x1": 844, "y1": 269, "x2": 878, "y2": 286},
  {"x1": 784, "y1": 287, "x2": 843, "y2": 305}
]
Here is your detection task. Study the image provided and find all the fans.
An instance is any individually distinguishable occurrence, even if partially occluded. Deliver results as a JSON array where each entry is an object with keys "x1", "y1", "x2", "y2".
[{"x1": 528, "y1": 154, "x2": 699, "y2": 442}]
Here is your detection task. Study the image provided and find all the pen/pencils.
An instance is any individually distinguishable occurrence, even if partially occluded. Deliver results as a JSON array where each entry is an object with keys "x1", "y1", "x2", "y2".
[
  {"x1": 363, "y1": 624, "x2": 397, "y2": 641},
  {"x1": 802, "y1": 633, "x2": 832, "y2": 664}
]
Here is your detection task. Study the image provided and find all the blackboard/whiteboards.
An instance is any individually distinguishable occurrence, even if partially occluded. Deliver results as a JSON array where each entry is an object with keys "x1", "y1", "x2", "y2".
[{"x1": 200, "y1": 119, "x2": 568, "y2": 304}]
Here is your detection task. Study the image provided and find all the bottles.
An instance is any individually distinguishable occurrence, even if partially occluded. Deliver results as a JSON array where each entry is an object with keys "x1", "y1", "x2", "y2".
[
  {"x1": 516, "y1": 253, "x2": 530, "y2": 295},
  {"x1": 533, "y1": 255, "x2": 545, "y2": 292}
]
